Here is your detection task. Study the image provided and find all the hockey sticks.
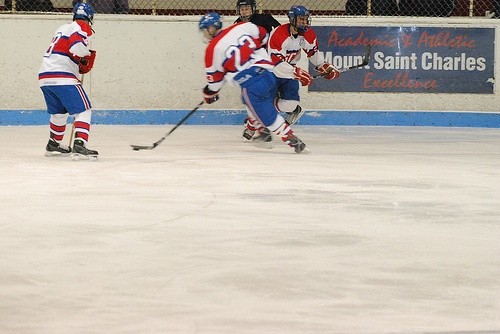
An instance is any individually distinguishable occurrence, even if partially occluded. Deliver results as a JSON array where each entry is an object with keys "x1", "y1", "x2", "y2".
[
  {"x1": 130, "y1": 99, "x2": 206, "y2": 150},
  {"x1": 69, "y1": 74, "x2": 84, "y2": 145},
  {"x1": 310, "y1": 44, "x2": 372, "y2": 79}
]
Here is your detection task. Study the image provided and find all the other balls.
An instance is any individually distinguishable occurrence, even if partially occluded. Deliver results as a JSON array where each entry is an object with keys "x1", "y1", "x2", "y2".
[{"x1": 133, "y1": 148, "x2": 139, "y2": 150}]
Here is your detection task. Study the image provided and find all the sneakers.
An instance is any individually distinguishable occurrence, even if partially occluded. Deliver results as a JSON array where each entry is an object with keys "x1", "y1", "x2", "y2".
[
  {"x1": 71, "y1": 139, "x2": 100, "y2": 161},
  {"x1": 45, "y1": 137, "x2": 72, "y2": 157},
  {"x1": 259, "y1": 128, "x2": 274, "y2": 145},
  {"x1": 272, "y1": 121, "x2": 309, "y2": 153},
  {"x1": 242, "y1": 117, "x2": 261, "y2": 143}
]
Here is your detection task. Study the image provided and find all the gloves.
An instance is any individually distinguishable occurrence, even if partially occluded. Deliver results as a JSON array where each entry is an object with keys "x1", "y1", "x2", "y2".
[
  {"x1": 313, "y1": 61, "x2": 340, "y2": 80},
  {"x1": 79, "y1": 50, "x2": 96, "y2": 74},
  {"x1": 292, "y1": 65, "x2": 313, "y2": 86},
  {"x1": 203, "y1": 85, "x2": 219, "y2": 104}
]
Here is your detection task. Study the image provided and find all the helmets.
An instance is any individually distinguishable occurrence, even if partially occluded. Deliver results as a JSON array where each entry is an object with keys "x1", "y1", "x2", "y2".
[
  {"x1": 198, "y1": 13, "x2": 222, "y2": 29},
  {"x1": 71, "y1": 2, "x2": 94, "y2": 20},
  {"x1": 236, "y1": 0, "x2": 257, "y2": 6},
  {"x1": 288, "y1": 6, "x2": 309, "y2": 21}
]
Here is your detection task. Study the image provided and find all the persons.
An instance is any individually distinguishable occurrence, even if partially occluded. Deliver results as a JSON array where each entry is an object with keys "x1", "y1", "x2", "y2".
[
  {"x1": 232, "y1": 0, "x2": 282, "y2": 52},
  {"x1": 243, "y1": 6, "x2": 340, "y2": 142},
  {"x1": 37, "y1": 3, "x2": 99, "y2": 158},
  {"x1": 199, "y1": 13, "x2": 305, "y2": 154}
]
